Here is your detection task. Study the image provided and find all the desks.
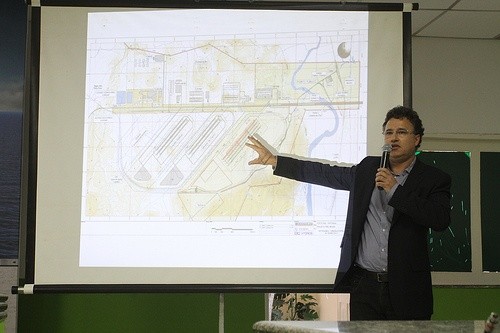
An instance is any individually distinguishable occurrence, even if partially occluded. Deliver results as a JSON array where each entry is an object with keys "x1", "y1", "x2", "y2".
[{"x1": 254, "y1": 318, "x2": 500, "y2": 333}]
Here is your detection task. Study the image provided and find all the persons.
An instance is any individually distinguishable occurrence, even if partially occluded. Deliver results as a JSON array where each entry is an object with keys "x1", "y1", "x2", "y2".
[{"x1": 244, "y1": 104, "x2": 451, "y2": 322}]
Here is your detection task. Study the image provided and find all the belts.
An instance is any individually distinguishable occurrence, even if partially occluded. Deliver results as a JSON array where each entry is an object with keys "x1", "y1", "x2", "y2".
[{"x1": 350, "y1": 266, "x2": 389, "y2": 284}]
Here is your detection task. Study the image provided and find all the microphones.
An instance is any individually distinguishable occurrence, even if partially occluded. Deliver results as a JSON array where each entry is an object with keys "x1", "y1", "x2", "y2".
[{"x1": 378, "y1": 143, "x2": 393, "y2": 190}]
